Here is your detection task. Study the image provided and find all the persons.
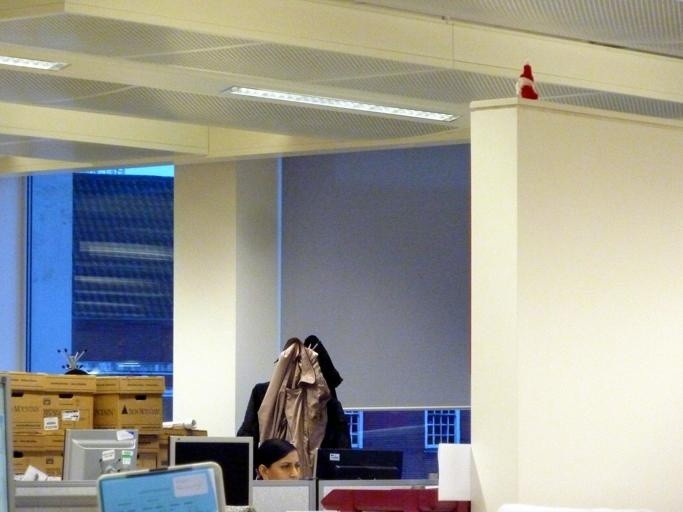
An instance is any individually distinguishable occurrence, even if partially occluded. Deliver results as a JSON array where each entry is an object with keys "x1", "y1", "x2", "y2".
[{"x1": 252, "y1": 439, "x2": 300, "y2": 480}]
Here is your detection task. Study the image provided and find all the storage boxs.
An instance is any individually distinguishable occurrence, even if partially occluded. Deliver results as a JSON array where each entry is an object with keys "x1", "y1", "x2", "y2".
[{"x1": 0, "y1": 370, "x2": 207, "y2": 483}]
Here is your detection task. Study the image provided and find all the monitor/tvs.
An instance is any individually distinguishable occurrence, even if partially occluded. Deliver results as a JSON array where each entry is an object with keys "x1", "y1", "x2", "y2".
[
  {"x1": 95, "y1": 461, "x2": 227, "y2": 512},
  {"x1": 62, "y1": 428, "x2": 139, "y2": 480},
  {"x1": 167, "y1": 435, "x2": 258, "y2": 512},
  {"x1": 312, "y1": 447, "x2": 404, "y2": 482}
]
